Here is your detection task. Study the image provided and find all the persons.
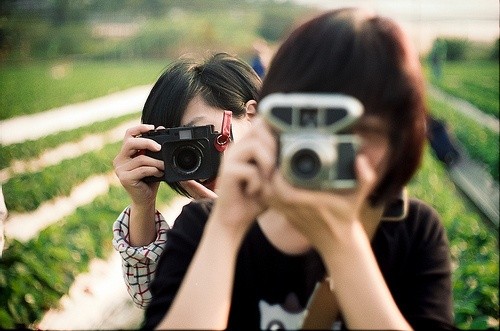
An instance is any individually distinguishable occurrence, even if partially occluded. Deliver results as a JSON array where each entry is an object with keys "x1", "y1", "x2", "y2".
[
  {"x1": 109, "y1": 50, "x2": 264, "y2": 311},
  {"x1": 134, "y1": 3, "x2": 462, "y2": 331}
]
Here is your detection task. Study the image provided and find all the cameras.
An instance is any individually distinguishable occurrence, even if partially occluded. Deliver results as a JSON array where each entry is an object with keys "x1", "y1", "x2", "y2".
[
  {"x1": 140, "y1": 125, "x2": 221, "y2": 184},
  {"x1": 257, "y1": 93, "x2": 363, "y2": 191}
]
